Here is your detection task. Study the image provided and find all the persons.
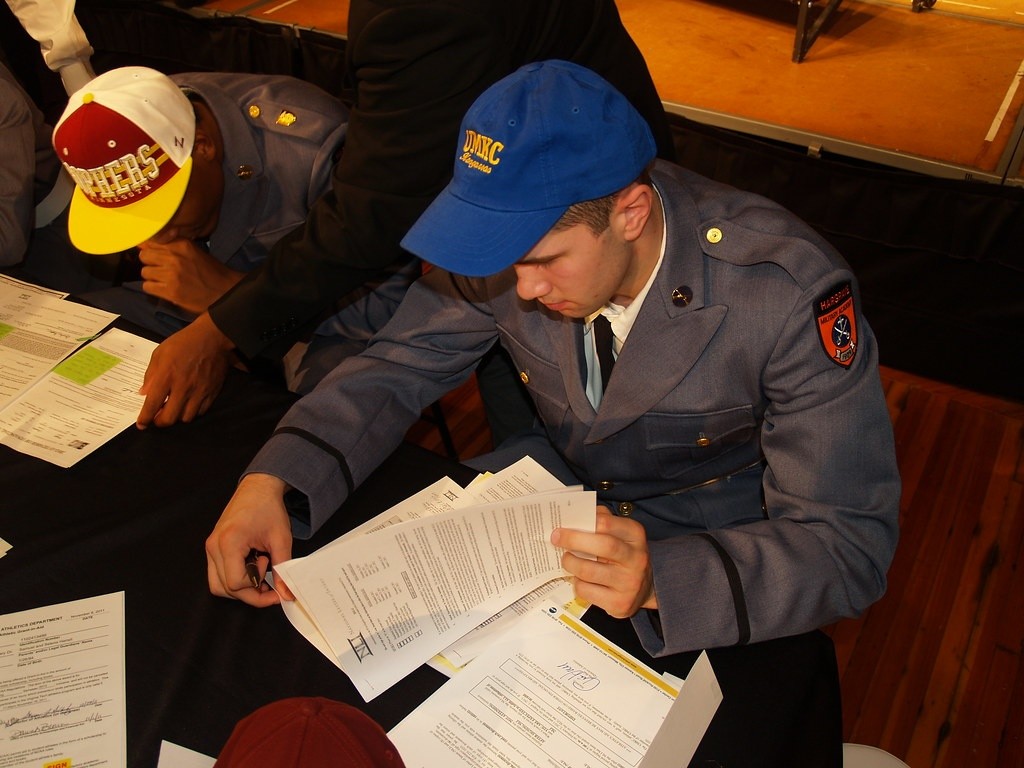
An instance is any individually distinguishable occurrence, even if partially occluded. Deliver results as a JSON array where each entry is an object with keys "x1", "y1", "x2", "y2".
[
  {"x1": 132, "y1": 0, "x2": 670, "y2": 454},
  {"x1": 214, "y1": 694, "x2": 408, "y2": 768},
  {"x1": 205, "y1": 56, "x2": 904, "y2": 767},
  {"x1": 45, "y1": 60, "x2": 425, "y2": 399},
  {"x1": 0, "y1": 61, "x2": 64, "y2": 287},
  {"x1": 2, "y1": 0, "x2": 100, "y2": 101}
]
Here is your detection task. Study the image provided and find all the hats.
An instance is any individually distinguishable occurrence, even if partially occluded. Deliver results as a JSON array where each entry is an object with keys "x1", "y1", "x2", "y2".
[
  {"x1": 52, "y1": 65, "x2": 196, "y2": 255},
  {"x1": 212, "y1": 696, "x2": 409, "y2": 768},
  {"x1": 399, "y1": 59, "x2": 658, "y2": 278}
]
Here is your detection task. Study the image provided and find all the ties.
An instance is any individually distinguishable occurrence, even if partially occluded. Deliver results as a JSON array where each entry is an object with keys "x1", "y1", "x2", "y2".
[{"x1": 593, "y1": 316, "x2": 616, "y2": 401}]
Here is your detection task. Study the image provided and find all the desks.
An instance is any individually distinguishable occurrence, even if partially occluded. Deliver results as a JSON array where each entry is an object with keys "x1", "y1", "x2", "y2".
[{"x1": 0, "y1": 273, "x2": 843, "y2": 768}]
